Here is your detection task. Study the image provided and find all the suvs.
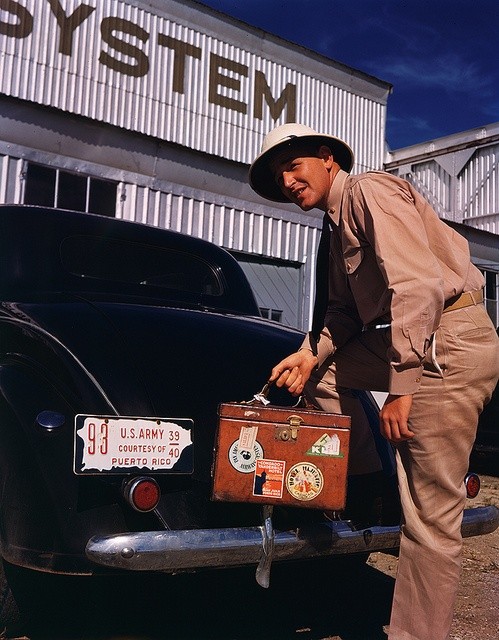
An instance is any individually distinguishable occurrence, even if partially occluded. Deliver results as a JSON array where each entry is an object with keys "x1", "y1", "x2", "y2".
[{"x1": 1, "y1": 203, "x2": 498, "y2": 640}]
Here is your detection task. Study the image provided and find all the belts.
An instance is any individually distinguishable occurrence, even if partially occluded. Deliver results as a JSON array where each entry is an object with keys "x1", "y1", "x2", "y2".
[{"x1": 441, "y1": 288, "x2": 486, "y2": 311}]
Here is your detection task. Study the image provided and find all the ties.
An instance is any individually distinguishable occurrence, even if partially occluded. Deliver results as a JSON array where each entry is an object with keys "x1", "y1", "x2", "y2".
[{"x1": 312, "y1": 214, "x2": 332, "y2": 342}]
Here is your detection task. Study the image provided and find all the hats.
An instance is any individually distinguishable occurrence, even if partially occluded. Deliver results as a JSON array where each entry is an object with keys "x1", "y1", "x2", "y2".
[{"x1": 249, "y1": 123, "x2": 354, "y2": 204}]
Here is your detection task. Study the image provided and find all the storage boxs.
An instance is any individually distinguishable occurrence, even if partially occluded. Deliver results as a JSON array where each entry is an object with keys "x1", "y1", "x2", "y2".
[{"x1": 209, "y1": 401, "x2": 351, "y2": 512}]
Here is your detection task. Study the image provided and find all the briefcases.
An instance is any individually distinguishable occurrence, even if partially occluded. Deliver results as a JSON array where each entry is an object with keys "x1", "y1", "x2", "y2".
[{"x1": 211, "y1": 377, "x2": 352, "y2": 510}]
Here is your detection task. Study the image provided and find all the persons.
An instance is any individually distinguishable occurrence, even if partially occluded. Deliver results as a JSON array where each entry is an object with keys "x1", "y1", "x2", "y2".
[{"x1": 249, "y1": 122, "x2": 499, "y2": 640}]
